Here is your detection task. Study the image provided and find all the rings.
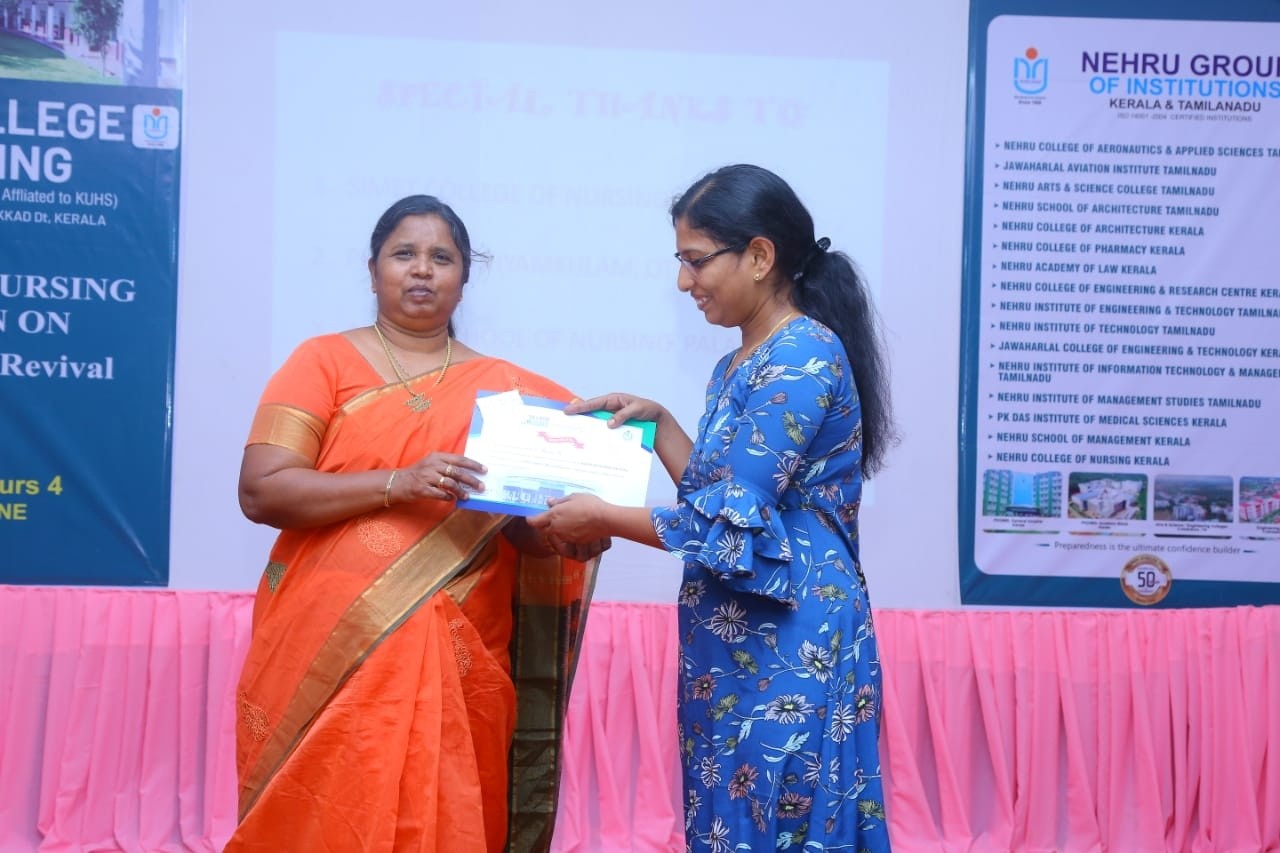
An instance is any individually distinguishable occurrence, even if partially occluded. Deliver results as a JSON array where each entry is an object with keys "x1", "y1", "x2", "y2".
[
  {"x1": 440, "y1": 476, "x2": 446, "y2": 488},
  {"x1": 446, "y1": 464, "x2": 452, "y2": 476}
]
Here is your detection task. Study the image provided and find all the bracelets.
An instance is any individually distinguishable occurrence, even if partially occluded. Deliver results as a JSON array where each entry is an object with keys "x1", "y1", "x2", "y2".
[{"x1": 385, "y1": 471, "x2": 396, "y2": 508}]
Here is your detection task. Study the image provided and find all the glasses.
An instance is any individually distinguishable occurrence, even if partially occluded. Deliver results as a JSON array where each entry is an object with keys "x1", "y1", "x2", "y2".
[{"x1": 673, "y1": 247, "x2": 732, "y2": 271}]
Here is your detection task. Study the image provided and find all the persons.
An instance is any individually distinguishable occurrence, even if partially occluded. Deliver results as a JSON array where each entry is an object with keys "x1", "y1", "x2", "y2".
[
  {"x1": 529, "y1": 162, "x2": 891, "y2": 853},
  {"x1": 238, "y1": 195, "x2": 609, "y2": 853}
]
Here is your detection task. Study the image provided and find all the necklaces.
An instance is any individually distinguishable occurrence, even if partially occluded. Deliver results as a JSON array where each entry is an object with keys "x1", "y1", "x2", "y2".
[
  {"x1": 727, "y1": 306, "x2": 798, "y2": 373},
  {"x1": 374, "y1": 322, "x2": 451, "y2": 397}
]
